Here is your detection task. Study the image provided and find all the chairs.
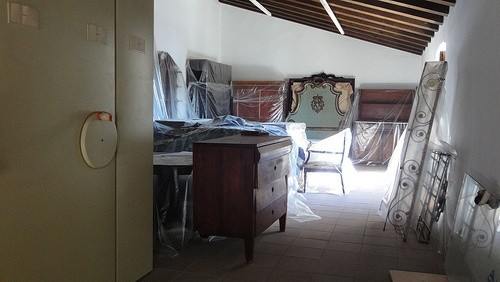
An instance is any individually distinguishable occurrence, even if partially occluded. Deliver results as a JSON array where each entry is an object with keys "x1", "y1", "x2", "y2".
[{"x1": 302, "y1": 130, "x2": 347, "y2": 195}]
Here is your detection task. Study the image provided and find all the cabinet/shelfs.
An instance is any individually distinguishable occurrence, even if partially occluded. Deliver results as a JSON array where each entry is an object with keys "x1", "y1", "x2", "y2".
[
  {"x1": 351, "y1": 88, "x2": 415, "y2": 169},
  {"x1": 231, "y1": 80, "x2": 288, "y2": 124},
  {"x1": 192, "y1": 135, "x2": 294, "y2": 265}
]
